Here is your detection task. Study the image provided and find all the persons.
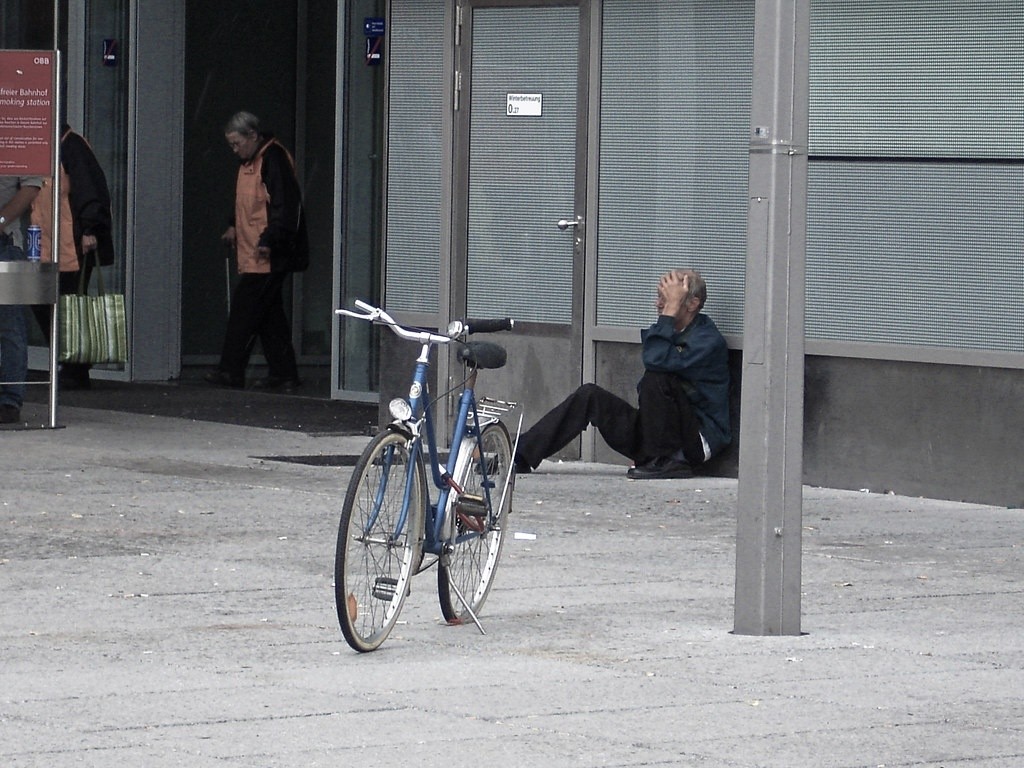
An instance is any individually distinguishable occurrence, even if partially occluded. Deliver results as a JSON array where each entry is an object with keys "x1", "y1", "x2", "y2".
[
  {"x1": 27, "y1": 120, "x2": 116, "y2": 390},
  {"x1": 203, "y1": 110, "x2": 311, "y2": 395},
  {"x1": 0, "y1": 174, "x2": 43, "y2": 424},
  {"x1": 474, "y1": 269, "x2": 733, "y2": 480}
]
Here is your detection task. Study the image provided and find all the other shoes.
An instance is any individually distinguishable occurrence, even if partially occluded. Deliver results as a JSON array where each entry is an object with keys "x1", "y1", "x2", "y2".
[
  {"x1": 474, "y1": 452, "x2": 531, "y2": 473},
  {"x1": 74, "y1": 371, "x2": 92, "y2": 391},
  {"x1": 627, "y1": 455, "x2": 693, "y2": 478},
  {"x1": 205, "y1": 371, "x2": 245, "y2": 389},
  {"x1": 0, "y1": 405, "x2": 19, "y2": 423},
  {"x1": 254, "y1": 375, "x2": 302, "y2": 394},
  {"x1": 45, "y1": 362, "x2": 74, "y2": 391}
]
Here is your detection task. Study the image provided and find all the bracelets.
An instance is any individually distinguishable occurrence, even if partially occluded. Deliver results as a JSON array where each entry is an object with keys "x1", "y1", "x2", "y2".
[{"x1": 0, "y1": 215, "x2": 9, "y2": 226}]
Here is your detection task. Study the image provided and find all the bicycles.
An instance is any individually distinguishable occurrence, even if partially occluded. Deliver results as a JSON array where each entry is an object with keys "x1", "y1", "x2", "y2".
[{"x1": 332, "y1": 300, "x2": 527, "y2": 654}]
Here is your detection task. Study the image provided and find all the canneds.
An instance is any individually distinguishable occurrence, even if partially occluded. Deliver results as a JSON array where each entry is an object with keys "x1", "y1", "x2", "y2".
[{"x1": 26, "y1": 225, "x2": 41, "y2": 262}]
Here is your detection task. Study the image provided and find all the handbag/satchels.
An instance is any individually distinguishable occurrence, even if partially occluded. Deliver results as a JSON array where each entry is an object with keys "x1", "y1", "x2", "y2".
[{"x1": 59, "y1": 248, "x2": 127, "y2": 363}]
